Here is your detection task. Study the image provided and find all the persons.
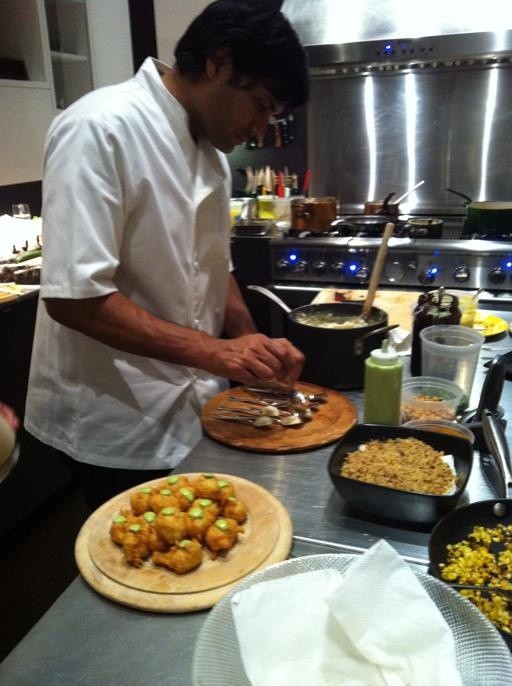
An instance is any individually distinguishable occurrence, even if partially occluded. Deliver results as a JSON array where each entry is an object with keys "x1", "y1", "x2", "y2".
[{"x1": 22, "y1": 1, "x2": 310, "y2": 515}]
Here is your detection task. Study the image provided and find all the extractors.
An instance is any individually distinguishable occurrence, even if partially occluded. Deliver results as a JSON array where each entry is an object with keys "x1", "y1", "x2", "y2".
[{"x1": 279, "y1": 0, "x2": 512, "y2": 78}]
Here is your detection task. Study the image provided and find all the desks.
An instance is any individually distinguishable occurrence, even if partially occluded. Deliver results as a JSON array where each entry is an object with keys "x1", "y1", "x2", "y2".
[{"x1": 1, "y1": 283, "x2": 511, "y2": 686}]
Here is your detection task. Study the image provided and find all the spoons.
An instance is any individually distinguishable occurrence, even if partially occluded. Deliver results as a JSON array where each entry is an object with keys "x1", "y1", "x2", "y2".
[{"x1": 212, "y1": 382, "x2": 327, "y2": 428}]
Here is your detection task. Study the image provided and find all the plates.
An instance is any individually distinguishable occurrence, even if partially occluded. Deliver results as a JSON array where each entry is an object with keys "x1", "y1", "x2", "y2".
[
  {"x1": 190, "y1": 552, "x2": 512, "y2": 686},
  {"x1": 472, "y1": 313, "x2": 509, "y2": 337},
  {"x1": 72, "y1": 472, "x2": 294, "y2": 613}
]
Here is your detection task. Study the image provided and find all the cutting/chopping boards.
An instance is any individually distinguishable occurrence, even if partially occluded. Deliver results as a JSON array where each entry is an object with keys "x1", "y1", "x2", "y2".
[{"x1": 200, "y1": 381, "x2": 358, "y2": 454}]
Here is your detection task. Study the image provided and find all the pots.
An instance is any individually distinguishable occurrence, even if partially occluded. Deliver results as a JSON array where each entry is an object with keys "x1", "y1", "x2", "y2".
[
  {"x1": 427, "y1": 409, "x2": 511, "y2": 637},
  {"x1": 246, "y1": 284, "x2": 389, "y2": 391},
  {"x1": 296, "y1": 180, "x2": 512, "y2": 241}
]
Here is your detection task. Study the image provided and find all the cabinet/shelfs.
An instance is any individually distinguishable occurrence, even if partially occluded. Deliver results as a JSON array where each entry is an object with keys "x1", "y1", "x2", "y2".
[{"x1": 1, "y1": 0, "x2": 93, "y2": 116}]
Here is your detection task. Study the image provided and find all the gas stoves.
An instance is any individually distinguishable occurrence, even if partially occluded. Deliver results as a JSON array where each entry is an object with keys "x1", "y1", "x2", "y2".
[{"x1": 266, "y1": 213, "x2": 512, "y2": 292}]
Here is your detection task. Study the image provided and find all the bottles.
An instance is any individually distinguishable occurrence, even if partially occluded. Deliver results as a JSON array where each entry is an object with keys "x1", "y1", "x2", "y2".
[{"x1": 363, "y1": 338, "x2": 404, "y2": 426}]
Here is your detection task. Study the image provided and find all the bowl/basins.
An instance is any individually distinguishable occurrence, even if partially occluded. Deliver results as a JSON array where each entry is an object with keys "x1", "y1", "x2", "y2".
[{"x1": 325, "y1": 423, "x2": 474, "y2": 527}]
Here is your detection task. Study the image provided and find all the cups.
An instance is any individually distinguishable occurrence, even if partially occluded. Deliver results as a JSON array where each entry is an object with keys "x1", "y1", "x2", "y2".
[
  {"x1": 12, "y1": 203, "x2": 31, "y2": 219},
  {"x1": 229, "y1": 195, "x2": 302, "y2": 236},
  {"x1": 397, "y1": 290, "x2": 486, "y2": 447}
]
